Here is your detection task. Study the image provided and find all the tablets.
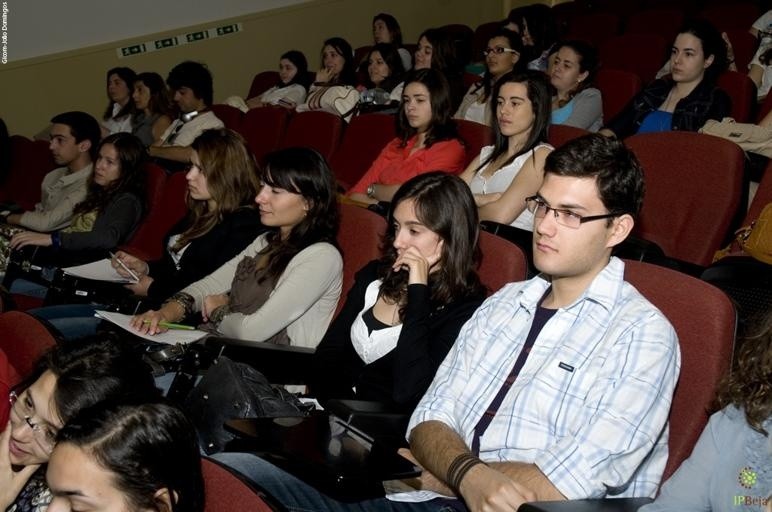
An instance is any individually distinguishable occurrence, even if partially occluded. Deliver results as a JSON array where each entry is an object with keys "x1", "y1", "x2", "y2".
[{"x1": 224, "y1": 414, "x2": 422, "y2": 482}]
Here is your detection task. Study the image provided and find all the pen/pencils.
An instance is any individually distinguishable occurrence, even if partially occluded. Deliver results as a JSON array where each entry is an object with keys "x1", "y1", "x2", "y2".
[
  {"x1": 108, "y1": 250, "x2": 140, "y2": 281},
  {"x1": 143, "y1": 319, "x2": 195, "y2": 331}
]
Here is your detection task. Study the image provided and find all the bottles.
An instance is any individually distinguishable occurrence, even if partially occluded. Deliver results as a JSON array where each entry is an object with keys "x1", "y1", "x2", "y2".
[{"x1": 360, "y1": 88, "x2": 390, "y2": 105}]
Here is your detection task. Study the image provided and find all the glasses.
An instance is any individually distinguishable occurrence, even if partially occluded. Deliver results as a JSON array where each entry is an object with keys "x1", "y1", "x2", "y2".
[
  {"x1": 483, "y1": 47, "x2": 521, "y2": 56},
  {"x1": 525, "y1": 195, "x2": 622, "y2": 229},
  {"x1": 9, "y1": 384, "x2": 59, "y2": 456}
]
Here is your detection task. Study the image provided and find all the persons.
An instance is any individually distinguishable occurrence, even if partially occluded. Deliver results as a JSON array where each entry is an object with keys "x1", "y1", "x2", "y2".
[{"x1": 0, "y1": 1, "x2": 772, "y2": 511}]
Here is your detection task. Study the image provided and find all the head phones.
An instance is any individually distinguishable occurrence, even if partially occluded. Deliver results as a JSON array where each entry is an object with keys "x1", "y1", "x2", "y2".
[{"x1": 182, "y1": 107, "x2": 209, "y2": 122}]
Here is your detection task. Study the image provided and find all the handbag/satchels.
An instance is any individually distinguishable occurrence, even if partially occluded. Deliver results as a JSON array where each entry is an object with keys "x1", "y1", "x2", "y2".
[
  {"x1": 137, "y1": 342, "x2": 209, "y2": 377},
  {"x1": 0, "y1": 223, "x2": 40, "y2": 272},
  {"x1": 736, "y1": 204, "x2": 771, "y2": 266},
  {"x1": 183, "y1": 356, "x2": 316, "y2": 452}
]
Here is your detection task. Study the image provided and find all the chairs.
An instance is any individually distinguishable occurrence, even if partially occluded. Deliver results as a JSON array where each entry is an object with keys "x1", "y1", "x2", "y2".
[{"x1": 0, "y1": 11, "x2": 771, "y2": 512}]
